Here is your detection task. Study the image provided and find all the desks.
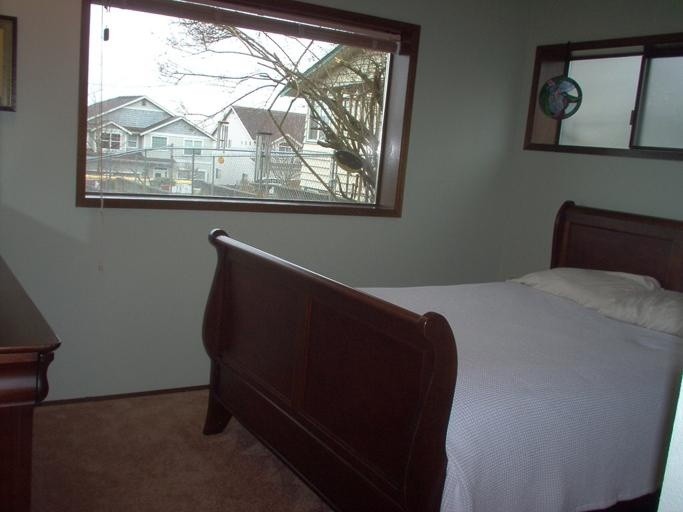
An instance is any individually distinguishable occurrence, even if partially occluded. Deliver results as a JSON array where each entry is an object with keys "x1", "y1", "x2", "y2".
[{"x1": 0, "y1": 252, "x2": 63, "y2": 512}]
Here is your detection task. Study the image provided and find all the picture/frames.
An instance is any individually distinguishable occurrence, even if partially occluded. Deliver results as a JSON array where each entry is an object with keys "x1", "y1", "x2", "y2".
[{"x1": 0, "y1": 14, "x2": 18, "y2": 112}]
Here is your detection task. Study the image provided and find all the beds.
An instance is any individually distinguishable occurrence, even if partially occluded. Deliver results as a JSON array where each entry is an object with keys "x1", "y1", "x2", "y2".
[{"x1": 200, "y1": 200, "x2": 683, "y2": 512}]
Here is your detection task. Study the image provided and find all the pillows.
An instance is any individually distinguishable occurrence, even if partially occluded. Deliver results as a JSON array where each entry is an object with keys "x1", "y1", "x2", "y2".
[
  {"x1": 599, "y1": 288, "x2": 683, "y2": 338},
  {"x1": 504, "y1": 264, "x2": 661, "y2": 318}
]
